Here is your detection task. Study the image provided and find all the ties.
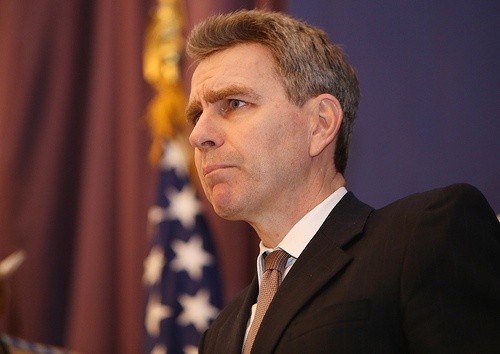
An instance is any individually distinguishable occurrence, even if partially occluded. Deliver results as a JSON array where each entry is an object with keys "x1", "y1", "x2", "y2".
[{"x1": 240, "y1": 251, "x2": 292, "y2": 354}]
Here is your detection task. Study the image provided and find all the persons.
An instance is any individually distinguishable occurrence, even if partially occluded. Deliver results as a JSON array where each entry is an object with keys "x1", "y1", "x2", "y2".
[{"x1": 186, "y1": 10, "x2": 500, "y2": 354}]
